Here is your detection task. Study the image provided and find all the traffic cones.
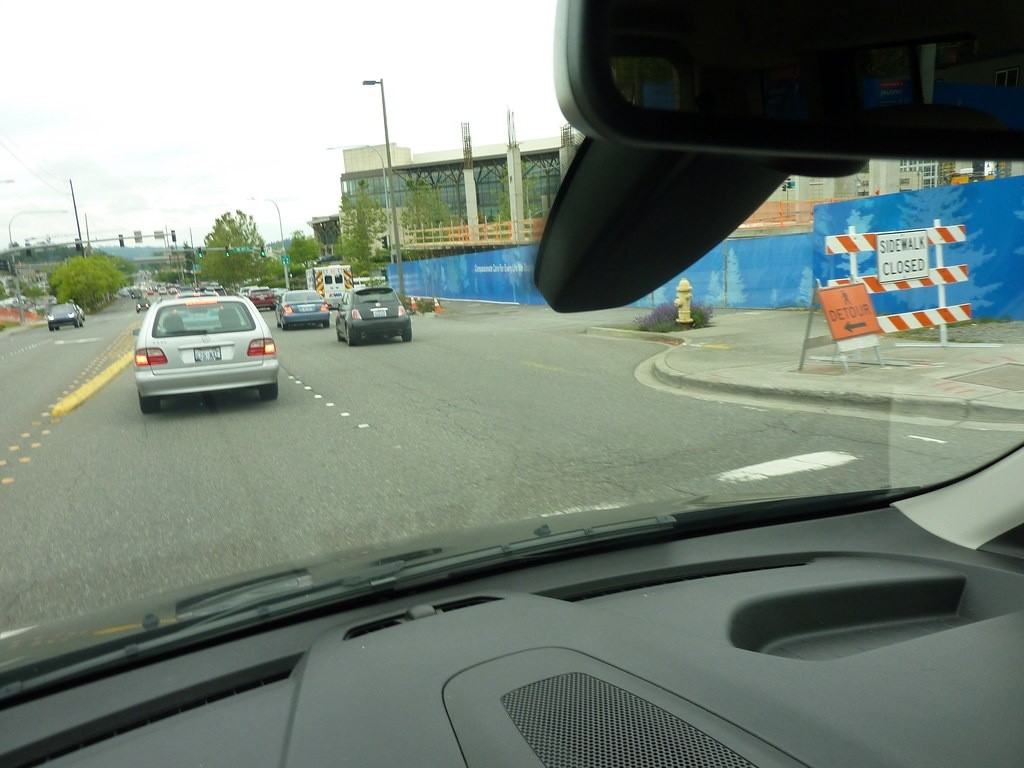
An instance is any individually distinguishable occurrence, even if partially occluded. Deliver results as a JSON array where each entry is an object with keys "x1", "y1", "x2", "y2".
[
  {"x1": 410, "y1": 296, "x2": 418, "y2": 310},
  {"x1": 434, "y1": 298, "x2": 443, "y2": 314}
]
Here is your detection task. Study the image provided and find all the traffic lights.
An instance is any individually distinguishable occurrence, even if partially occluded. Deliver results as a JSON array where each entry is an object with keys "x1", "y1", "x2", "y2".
[
  {"x1": 225, "y1": 246, "x2": 229, "y2": 256},
  {"x1": 261, "y1": 247, "x2": 264, "y2": 256},
  {"x1": 198, "y1": 248, "x2": 203, "y2": 258}
]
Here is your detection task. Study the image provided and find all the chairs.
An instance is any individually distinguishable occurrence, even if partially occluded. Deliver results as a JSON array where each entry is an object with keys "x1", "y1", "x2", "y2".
[
  {"x1": 162, "y1": 314, "x2": 185, "y2": 333},
  {"x1": 218, "y1": 308, "x2": 243, "y2": 328}
]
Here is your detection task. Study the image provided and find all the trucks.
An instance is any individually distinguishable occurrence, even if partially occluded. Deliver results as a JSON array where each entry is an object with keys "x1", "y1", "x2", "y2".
[{"x1": 306, "y1": 265, "x2": 353, "y2": 309}]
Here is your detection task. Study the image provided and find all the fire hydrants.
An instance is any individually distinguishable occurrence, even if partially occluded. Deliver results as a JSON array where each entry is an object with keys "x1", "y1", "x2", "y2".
[{"x1": 674, "y1": 277, "x2": 694, "y2": 328}]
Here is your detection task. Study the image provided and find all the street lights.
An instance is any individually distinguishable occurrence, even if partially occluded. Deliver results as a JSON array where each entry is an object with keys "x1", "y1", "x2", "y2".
[
  {"x1": 326, "y1": 144, "x2": 394, "y2": 264},
  {"x1": 362, "y1": 79, "x2": 403, "y2": 300},
  {"x1": 247, "y1": 196, "x2": 290, "y2": 288},
  {"x1": 9, "y1": 209, "x2": 68, "y2": 324}
]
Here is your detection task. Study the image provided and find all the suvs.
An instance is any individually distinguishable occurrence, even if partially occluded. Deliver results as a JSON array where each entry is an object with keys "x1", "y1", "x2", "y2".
[{"x1": 245, "y1": 287, "x2": 276, "y2": 310}]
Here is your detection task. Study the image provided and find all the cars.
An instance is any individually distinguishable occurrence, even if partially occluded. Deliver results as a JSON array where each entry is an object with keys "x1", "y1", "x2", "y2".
[
  {"x1": 175, "y1": 286, "x2": 289, "y2": 298},
  {"x1": 275, "y1": 290, "x2": 329, "y2": 329},
  {"x1": 129, "y1": 282, "x2": 179, "y2": 314},
  {"x1": 334, "y1": 287, "x2": 412, "y2": 346},
  {"x1": 46, "y1": 303, "x2": 85, "y2": 331},
  {"x1": 132, "y1": 294, "x2": 281, "y2": 413}
]
